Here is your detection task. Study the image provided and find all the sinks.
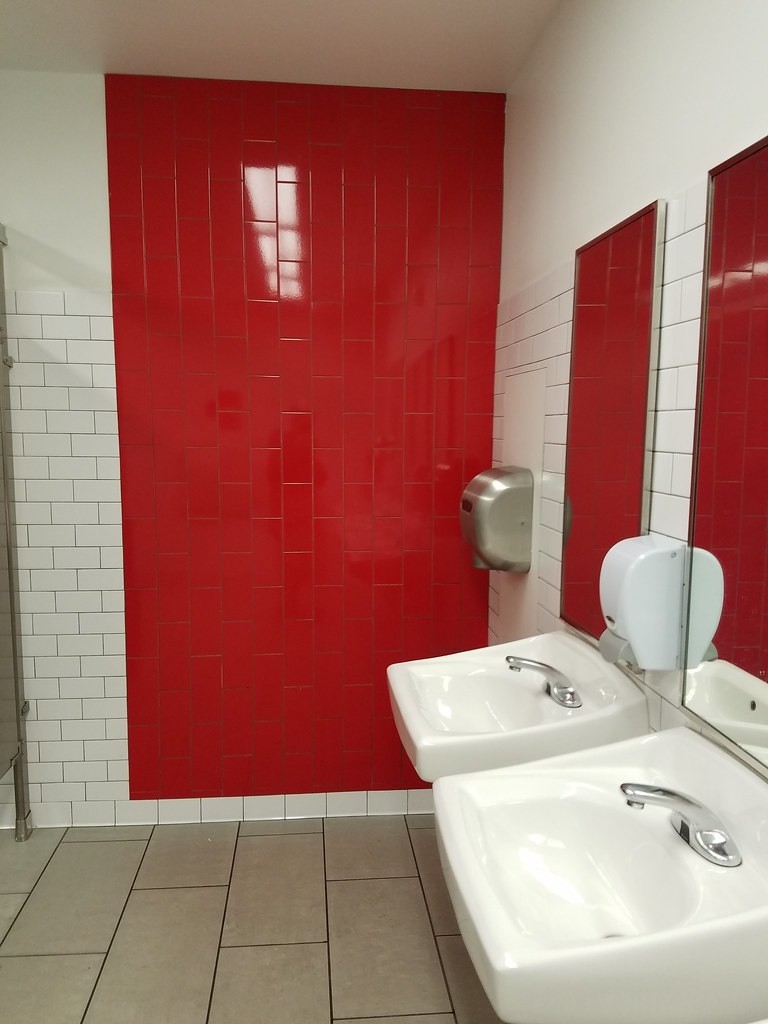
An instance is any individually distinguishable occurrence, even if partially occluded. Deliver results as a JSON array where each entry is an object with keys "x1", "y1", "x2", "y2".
[
  {"x1": 386, "y1": 631, "x2": 649, "y2": 783},
  {"x1": 432, "y1": 726, "x2": 768, "y2": 1024},
  {"x1": 738, "y1": 743, "x2": 768, "y2": 767},
  {"x1": 686, "y1": 659, "x2": 768, "y2": 748}
]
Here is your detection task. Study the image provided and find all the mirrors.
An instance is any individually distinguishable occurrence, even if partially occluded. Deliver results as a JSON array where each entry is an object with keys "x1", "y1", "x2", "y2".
[
  {"x1": 559, "y1": 198, "x2": 667, "y2": 675},
  {"x1": 676, "y1": 136, "x2": 768, "y2": 780}
]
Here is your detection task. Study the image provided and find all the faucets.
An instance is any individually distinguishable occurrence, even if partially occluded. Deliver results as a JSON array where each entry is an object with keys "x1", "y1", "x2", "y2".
[
  {"x1": 620, "y1": 783, "x2": 742, "y2": 867},
  {"x1": 505, "y1": 656, "x2": 582, "y2": 708}
]
